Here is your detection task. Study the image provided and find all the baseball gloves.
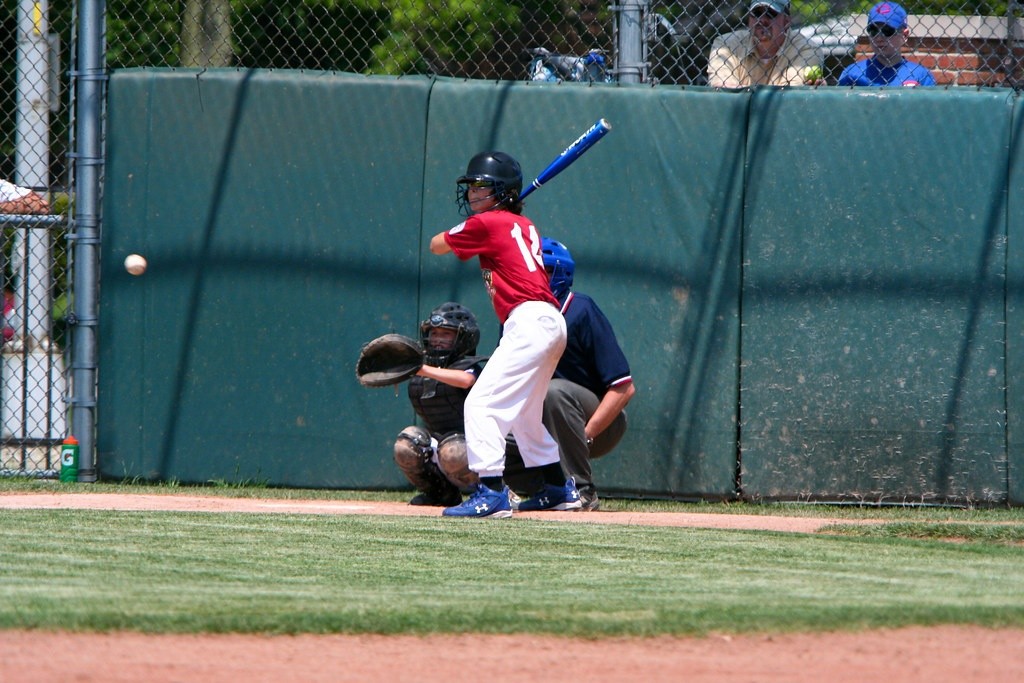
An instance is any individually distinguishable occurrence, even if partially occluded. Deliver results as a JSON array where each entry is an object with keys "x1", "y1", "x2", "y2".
[{"x1": 354, "y1": 328, "x2": 425, "y2": 398}]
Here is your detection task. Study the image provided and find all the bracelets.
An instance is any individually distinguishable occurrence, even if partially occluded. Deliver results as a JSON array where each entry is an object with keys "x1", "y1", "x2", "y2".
[{"x1": 587, "y1": 438, "x2": 593, "y2": 446}]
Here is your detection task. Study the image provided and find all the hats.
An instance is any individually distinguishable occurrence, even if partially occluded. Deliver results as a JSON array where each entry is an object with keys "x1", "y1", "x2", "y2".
[
  {"x1": 749, "y1": 0, "x2": 791, "y2": 17},
  {"x1": 868, "y1": 3, "x2": 908, "y2": 33}
]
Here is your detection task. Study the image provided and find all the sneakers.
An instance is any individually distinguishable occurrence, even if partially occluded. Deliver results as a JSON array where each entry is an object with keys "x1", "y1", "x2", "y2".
[
  {"x1": 518, "y1": 478, "x2": 582, "y2": 514},
  {"x1": 444, "y1": 482, "x2": 514, "y2": 520}
]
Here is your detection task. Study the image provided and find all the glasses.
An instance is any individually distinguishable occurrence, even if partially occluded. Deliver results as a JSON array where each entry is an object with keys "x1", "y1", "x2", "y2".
[
  {"x1": 869, "y1": 25, "x2": 896, "y2": 38},
  {"x1": 752, "y1": 7, "x2": 781, "y2": 19}
]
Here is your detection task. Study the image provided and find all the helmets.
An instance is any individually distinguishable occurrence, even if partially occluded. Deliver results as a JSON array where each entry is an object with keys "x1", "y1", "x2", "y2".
[
  {"x1": 420, "y1": 301, "x2": 480, "y2": 369},
  {"x1": 456, "y1": 151, "x2": 522, "y2": 215},
  {"x1": 539, "y1": 237, "x2": 576, "y2": 297}
]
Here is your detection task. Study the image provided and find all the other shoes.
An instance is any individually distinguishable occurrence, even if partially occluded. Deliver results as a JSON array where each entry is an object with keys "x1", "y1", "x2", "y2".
[
  {"x1": 412, "y1": 494, "x2": 462, "y2": 506},
  {"x1": 584, "y1": 487, "x2": 600, "y2": 513}
]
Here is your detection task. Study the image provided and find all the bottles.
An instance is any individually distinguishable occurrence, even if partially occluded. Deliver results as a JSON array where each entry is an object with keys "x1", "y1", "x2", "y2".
[{"x1": 60, "y1": 436, "x2": 79, "y2": 483}]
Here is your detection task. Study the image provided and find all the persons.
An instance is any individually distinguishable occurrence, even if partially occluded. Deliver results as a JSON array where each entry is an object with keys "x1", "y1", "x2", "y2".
[
  {"x1": 355, "y1": 301, "x2": 492, "y2": 508},
  {"x1": 707, "y1": 0, "x2": 824, "y2": 88},
  {"x1": 429, "y1": 150, "x2": 582, "y2": 519},
  {"x1": 806, "y1": 1, "x2": 937, "y2": 86},
  {"x1": 498, "y1": 235, "x2": 635, "y2": 511},
  {"x1": 0, "y1": 178, "x2": 50, "y2": 344}
]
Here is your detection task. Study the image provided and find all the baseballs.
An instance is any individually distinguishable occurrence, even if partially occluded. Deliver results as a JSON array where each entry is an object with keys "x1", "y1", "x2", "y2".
[{"x1": 124, "y1": 254, "x2": 147, "y2": 276}]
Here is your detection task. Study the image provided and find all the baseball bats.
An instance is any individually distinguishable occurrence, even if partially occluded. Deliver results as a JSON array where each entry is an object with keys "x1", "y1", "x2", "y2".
[{"x1": 519, "y1": 118, "x2": 612, "y2": 201}]
